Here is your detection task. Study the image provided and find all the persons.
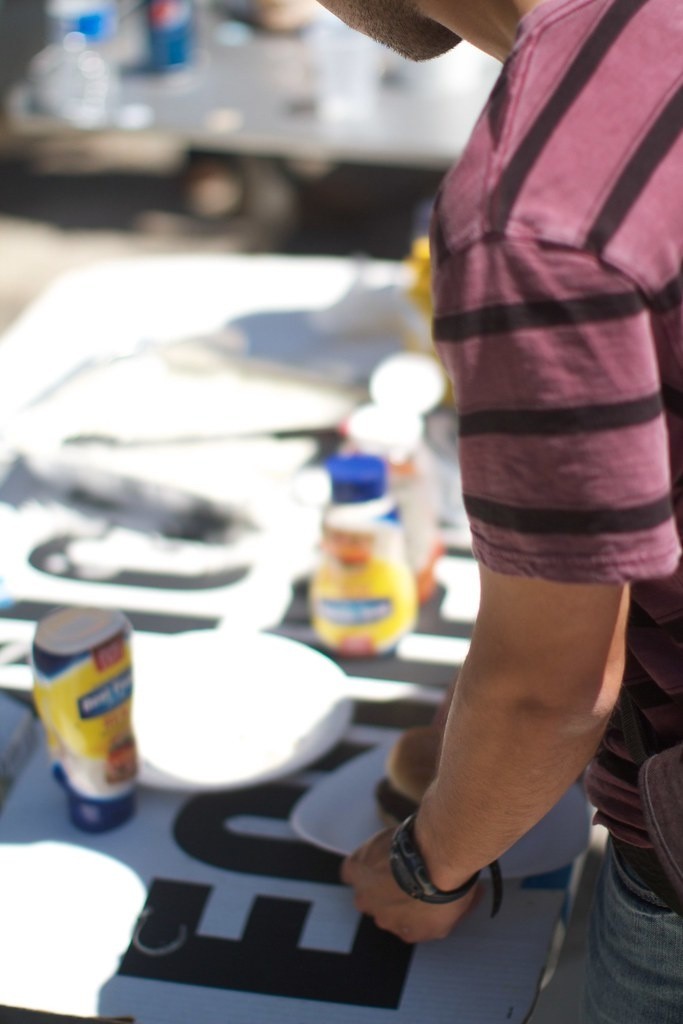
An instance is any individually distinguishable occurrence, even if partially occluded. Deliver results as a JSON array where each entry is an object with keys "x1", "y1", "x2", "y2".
[{"x1": 321, "y1": 1, "x2": 683, "y2": 1024}]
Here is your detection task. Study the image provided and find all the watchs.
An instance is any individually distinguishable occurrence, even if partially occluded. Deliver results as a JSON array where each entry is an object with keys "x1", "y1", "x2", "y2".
[{"x1": 390, "y1": 809, "x2": 502, "y2": 918}]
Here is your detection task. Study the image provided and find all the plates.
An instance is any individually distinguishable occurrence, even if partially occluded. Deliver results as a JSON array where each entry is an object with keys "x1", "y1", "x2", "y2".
[
  {"x1": 292, "y1": 747, "x2": 592, "y2": 883},
  {"x1": 131, "y1": 629, "x2": 355, "y2": 796}
]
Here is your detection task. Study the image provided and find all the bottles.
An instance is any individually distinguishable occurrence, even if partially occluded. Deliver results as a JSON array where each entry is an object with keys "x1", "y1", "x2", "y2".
[
  {"x1": 404, "y1": 236, "x2": 454, "y2": 404},
  {"x1": 310, "y1": 456, "x2": 417, "y2": 658},
  {"x1": 146, "y1": 0, "x2": 200, "y2": 71},
  {"x1": 30, "y1": 609, "x2": 138, "y2": 831},
  {"x1": 337, "y1": 410, "x2": 440, "y2": 607},
  {"x1": 39, "y1": 0, "x2": 117, "y2": 80}
]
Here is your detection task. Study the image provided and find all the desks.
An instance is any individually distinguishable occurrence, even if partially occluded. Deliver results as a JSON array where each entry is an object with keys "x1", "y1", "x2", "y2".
[{"x1": 0, "y1": 250, "x2": 589, "y2": 1024}]
